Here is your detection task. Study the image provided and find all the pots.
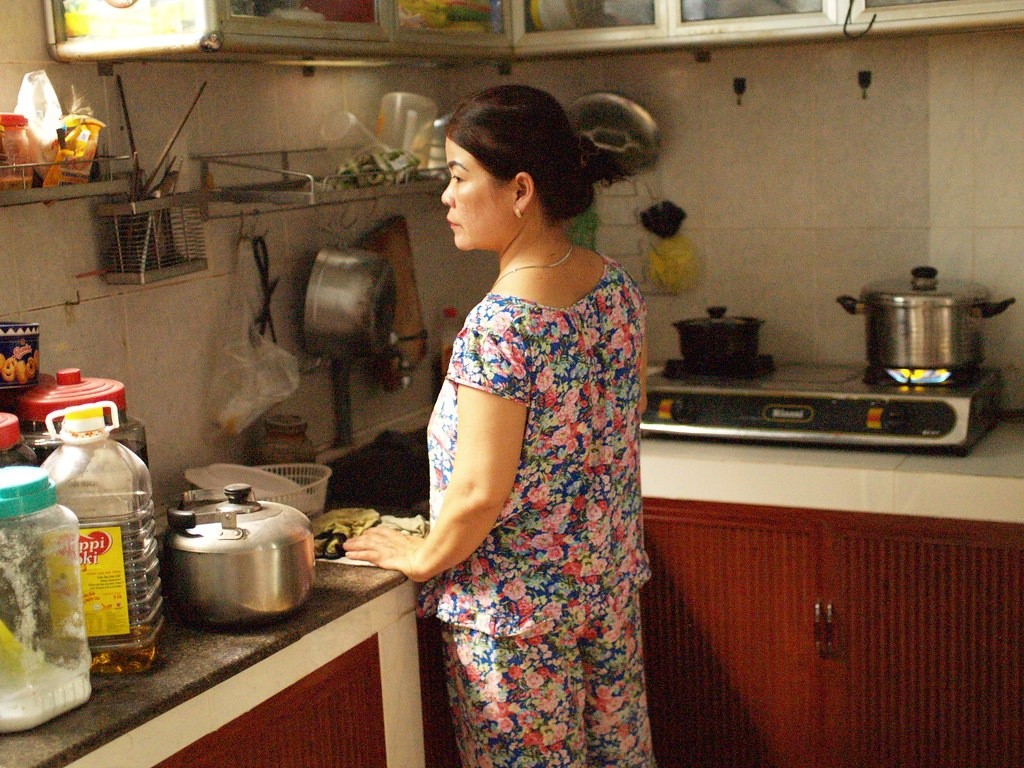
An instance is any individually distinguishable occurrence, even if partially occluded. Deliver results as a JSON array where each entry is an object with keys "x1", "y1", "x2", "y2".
[
  {"x1": 840, "y1": 268, "x2": 1014, "y2": 368},
  {"x1": 304, "y1": 250, "x2": 395, "y2": 447},
  {"x1": 673, "y1": 305, "x2": 761, "y2": 368}
]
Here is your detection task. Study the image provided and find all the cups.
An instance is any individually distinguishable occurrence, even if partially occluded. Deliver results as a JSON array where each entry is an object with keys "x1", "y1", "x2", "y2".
[
  {"x1": 379, "y1": 93, "x2": 435, "y2": 169},
  {"x1": 322, "y1": 109, "x2": 385, "y2": 169}
]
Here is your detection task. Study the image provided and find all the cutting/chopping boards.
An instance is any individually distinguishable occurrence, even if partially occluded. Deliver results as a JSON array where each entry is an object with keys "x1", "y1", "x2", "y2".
[{"x1": 348, "y1": 216, "x2": 428, "y2": 390}]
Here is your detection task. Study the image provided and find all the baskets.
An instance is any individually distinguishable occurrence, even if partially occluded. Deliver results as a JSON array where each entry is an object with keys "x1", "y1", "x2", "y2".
[{"x1": 251, "y1": 462, "x2": 332, "y2": 518}]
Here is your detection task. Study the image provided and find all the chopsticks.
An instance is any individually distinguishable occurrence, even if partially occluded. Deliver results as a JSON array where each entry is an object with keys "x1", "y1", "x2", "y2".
[{"x1": 106, "y1": 72, "x2": 213, "y2": 272}]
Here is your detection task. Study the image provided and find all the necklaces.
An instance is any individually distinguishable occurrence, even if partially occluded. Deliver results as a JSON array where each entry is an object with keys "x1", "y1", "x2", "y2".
[{"x1": 489, "y1": 241, "x2": 573, "y2": 290}]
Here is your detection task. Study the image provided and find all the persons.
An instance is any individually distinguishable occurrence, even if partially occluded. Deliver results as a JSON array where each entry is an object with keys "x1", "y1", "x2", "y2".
[{"x1": 343, "y1": 81, "x2": 657, "y2": 768}]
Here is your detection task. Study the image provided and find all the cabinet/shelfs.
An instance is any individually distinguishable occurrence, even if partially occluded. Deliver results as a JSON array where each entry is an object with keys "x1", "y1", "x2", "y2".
[
  {"x1": 512, "y1": 0, "x2": 851, "y2": 59},
  {"x1": 851, "y1": 0, "x2": 1024, "y2": 39},
  {"x1": 642, "y1": 496, "x2": 1024, "y2": 768},
  {"x1": 44, "y1": 0, "x2": 513, "y2": 63},
  {"x1": 62, "y1": 604, "x2": 463, "y2": 768}
]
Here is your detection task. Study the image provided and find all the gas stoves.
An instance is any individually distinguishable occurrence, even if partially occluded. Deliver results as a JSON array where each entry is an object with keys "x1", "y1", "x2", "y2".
[{"x1": 642, "y1": 357, "x2": 999, "y2": 457}]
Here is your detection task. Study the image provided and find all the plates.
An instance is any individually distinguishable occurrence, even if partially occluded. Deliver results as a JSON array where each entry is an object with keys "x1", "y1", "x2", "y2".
[{"x1": 184, "y1": 463, "x2": 298, "y2": 499}]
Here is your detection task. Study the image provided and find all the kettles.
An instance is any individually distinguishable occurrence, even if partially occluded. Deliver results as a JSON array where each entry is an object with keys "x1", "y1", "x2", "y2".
[{"x1": 168, "y1": 484, "x2": 316, "y2": 619}]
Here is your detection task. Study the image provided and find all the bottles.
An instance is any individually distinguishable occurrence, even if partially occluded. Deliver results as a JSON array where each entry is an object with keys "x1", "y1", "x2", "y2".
[
  {"x1": 0, "y1": 465, "x2": 92, "y2": 734},
  {"x1": 16, "y1": 367, "x2": 148, "y2": 481},
  {"x1": 0, "y1": 115, "x2": 33, "y2": 192},
  {"x1": 39, "y1": 401, "x2": 162, "y2": 681},
  {"x1": 440, "y1": 306, "x2": 459, "y2": 377},
  {"x1": 255, "y1": 415, "x2": 315, "y2": 464}
]
